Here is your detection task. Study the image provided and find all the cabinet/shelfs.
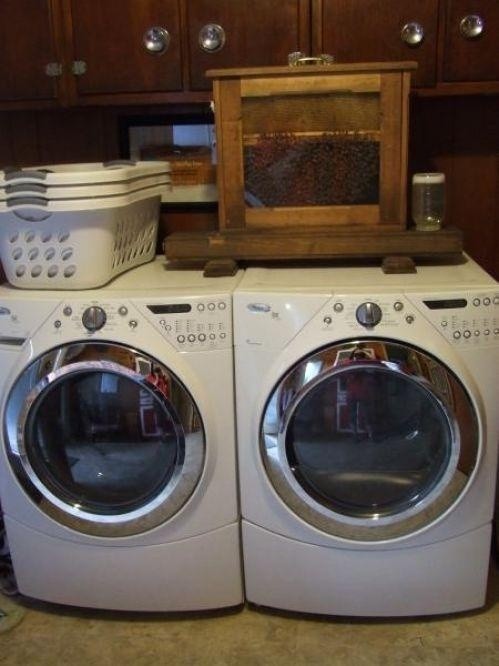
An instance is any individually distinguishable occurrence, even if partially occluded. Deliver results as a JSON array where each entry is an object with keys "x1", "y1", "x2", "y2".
[
  {"x1": 308, "y1": 0, "x2": 498, "y2": 98},
  {"x1": 1, "y1": 1, "x2": 59, "y2": 119},
  {"x1": 58, "y1": 0, "x2": 308, "y2": 112}
]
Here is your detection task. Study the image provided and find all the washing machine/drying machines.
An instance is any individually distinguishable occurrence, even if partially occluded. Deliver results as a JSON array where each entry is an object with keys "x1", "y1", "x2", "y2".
[
  {"x1": 233, "y1": 250, "x2": 499, "y2": 620},
  {"x1": 0, "y1": 253, "x2": 244, "y2": 613}
]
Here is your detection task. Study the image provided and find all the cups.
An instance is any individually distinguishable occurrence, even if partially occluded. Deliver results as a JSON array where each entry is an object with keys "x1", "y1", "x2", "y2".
[{"x1": 412, "y1": 172, "x2": 447, "y2": 232}]
